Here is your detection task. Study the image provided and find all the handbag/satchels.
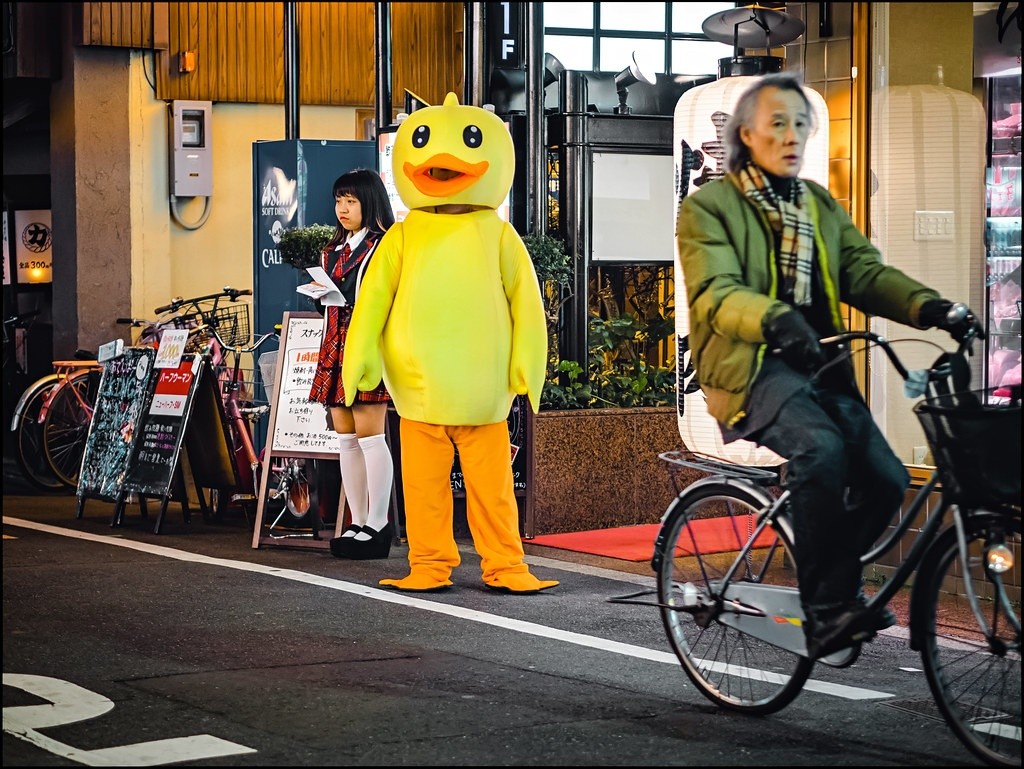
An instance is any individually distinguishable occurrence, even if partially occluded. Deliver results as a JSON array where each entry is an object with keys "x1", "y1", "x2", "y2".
[{"x1": 931, "y1": 353, "x2": 1024, "y2": 507}]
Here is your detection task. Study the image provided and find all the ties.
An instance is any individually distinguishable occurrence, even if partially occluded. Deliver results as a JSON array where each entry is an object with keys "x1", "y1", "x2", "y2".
[{"x1": 342, "y1": 243, "x2": 350, "y2": 262}]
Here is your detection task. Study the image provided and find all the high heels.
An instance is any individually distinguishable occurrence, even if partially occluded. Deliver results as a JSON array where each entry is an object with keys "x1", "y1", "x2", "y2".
[
  {"x1": 330, "y1": 524, "x2": 362, "y2": 557},
  {"x1": 342, "y1": 521, "x2": 392, "y2": 559}
]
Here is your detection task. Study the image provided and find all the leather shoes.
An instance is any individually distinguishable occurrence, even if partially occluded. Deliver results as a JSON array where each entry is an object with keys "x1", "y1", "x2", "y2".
[
  {"x1": 845, "y1": 595, "x2": 895, "y2": 631},
  {"x1": 803, "y1": 601, "x2": 866, "y2": 661}
]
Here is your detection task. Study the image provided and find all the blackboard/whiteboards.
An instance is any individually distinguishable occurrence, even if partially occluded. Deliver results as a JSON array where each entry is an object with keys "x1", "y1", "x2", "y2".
[
  {"x1": 76, "y1": 345, "x2": 196, "y2": 504},
  {"x1": 121, "y1": 352, "x2": 243, "y2": 498},
  {"x1": 270, "y1": 310, "x2": 392, "y2": 459}
]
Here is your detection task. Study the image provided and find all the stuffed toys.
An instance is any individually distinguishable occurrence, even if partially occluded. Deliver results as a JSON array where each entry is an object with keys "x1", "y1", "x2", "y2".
[{"x1": 341, "y1": 92, "x2": 560, "y2": 594}]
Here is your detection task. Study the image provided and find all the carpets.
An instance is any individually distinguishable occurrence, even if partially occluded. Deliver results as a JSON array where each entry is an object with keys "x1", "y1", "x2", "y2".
[{"x1": 521, "y1": 514, "x2": 784, "y2": 562}]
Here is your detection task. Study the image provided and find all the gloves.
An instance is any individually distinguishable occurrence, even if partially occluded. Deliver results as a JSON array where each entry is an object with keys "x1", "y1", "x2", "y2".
[
  {"x1": 762, "y1": 307, "x2": 824, "y2": 375},
  {"x1": 918, "y1": 299, "x2": 985, "y2": 357}
]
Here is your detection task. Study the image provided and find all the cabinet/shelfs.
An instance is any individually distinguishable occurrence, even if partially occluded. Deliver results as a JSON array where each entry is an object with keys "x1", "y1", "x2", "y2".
[{"x1": 983, "y1": 75, "x2": 1022, "y2": 406}]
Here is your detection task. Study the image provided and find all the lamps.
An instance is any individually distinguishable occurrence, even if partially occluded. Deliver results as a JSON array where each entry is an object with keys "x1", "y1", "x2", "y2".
[
  {"x1": 543, "y1": 53, "x2": 565, "y2": 111},
  {"x1": 613, "y1": 50, "x2": 656, "y2": 115}
]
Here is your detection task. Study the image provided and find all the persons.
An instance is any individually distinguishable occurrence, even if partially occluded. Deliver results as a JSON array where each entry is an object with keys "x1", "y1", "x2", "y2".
[
  {"x1": 308, "y1": 170, "x2": 394, "y2": 561},
  {"x1": 675, "y1": 73, "x2": 987, "y2": 661}
]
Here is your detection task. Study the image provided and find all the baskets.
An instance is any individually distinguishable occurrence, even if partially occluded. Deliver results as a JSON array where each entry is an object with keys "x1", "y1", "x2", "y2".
[
  {"x1": 202, "y1": 304, "x2": 250, "y2": 352},
  {"x1": 173, "y1": 311, "x2": 222, "y2": 353},
  {"x1": 258, "y1": 351, "x2": 278, "y2": 404},
  {"x1": 215, "y1": 366, "x2": 268, "y2": 403},
  {"x1": 912, "y1": 384, "x2": 1024, "y2": 504}
]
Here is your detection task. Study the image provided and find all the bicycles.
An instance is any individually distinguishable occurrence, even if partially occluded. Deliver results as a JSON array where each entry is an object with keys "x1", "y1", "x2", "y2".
[
  {"x1": 10, "y1": 286, "x2": 328, "y2": 542},
  {"x1": 605, "y1": 299, "x2": 1023, "y2": 768}
]
